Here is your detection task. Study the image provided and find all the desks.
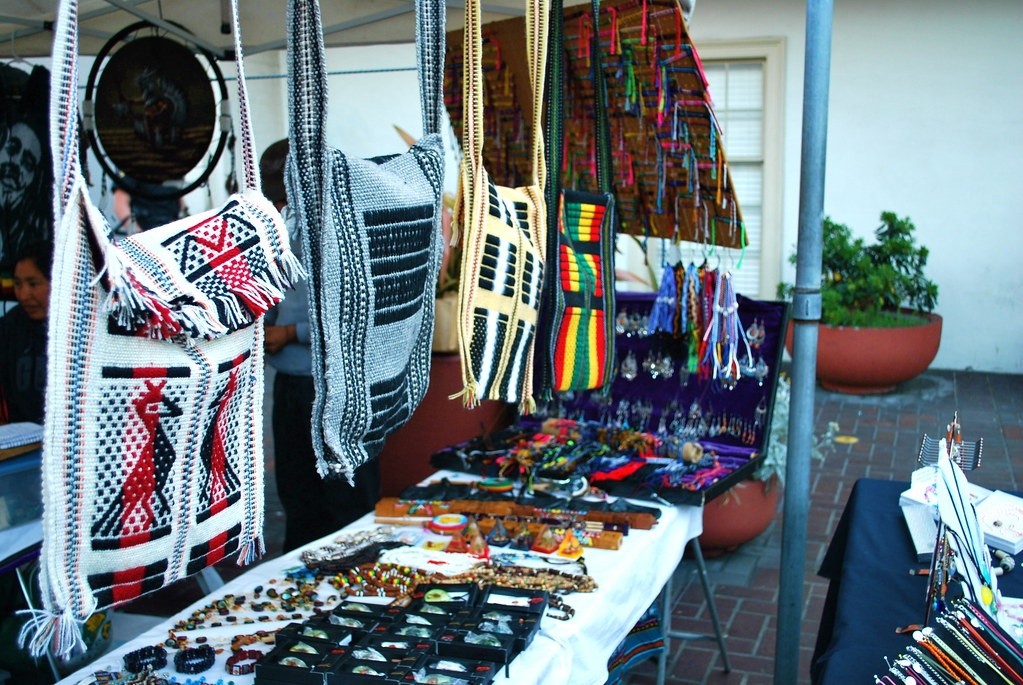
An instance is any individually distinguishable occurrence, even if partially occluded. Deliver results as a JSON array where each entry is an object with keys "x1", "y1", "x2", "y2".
[
  {"x1": 56, "y1": 465, "x2": 733, "y2": 685},
  {"x1": 809, "y1": 478, "x2": 1023, "y2": 685},
  {"x1": 0, "y1": 449, "x2": 61, "y2": 685}
]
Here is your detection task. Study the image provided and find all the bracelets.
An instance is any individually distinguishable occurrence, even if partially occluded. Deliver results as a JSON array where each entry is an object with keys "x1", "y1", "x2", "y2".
[
  {"x1": 225, "y1": 650, "x2": 265, "y2": 674},
  {"x1": 412, "y1": 609, "x2": 513, "y2": 685},
  {"x1": 122, "y1": 645, "x2": 168, "y2": 674},
  {"x1": 304, "y1": 630, "x2": 329, "y2": 640},
  {"x1": 381, "y1": 605, "x2": 446, "y2": 648},
  {"x1": 346, "y1": 603, "x2": 370, "y2": 612},
  {"x1": 344, "y1": 617, "x2": 363, "y2": 629},
  {"x1": 174, "y1": 644, "x2": 216, "y2": 675},
  {"x1": 360, "y1": 651, "x2": 386, "y2": 662},
  {"x1": 424, "y1": 588, "x2": 452, "y2": 602},
  {"x1": 352, "y1": 666, "x2": 373, "y2": 675},
  {"x1": 289, "y1": 643, "x2": 320, "y2": 654},
  {"x1": 281, "y1": 656, "x2": 306, "y2": 667}
]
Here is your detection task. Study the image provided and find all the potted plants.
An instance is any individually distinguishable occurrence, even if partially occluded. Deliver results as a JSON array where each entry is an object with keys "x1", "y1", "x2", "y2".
[
  {"x1": 777, "y1": 208, "x2": 941, "y2": 395},
  {"x1": 687, "y1": 372, "x2": 841, "y2": 561}
]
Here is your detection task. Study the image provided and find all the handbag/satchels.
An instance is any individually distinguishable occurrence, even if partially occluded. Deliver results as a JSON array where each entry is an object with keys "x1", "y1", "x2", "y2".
[
  {"x1": 439, "y1": 0, "x2": 551, "y2": 418},
  {"x1": 271, "y1": 0, "x2": 448, "y2": 487},
  {"x1": 544, "y1": 0, "x2": 615, "y2": 407},
  {"x1": 15, "y1": 0, "x2": 307, "y2": 661}
]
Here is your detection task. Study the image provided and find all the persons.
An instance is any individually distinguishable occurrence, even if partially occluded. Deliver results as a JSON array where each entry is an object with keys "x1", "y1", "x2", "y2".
[
  {"x1": 258, "y1": 139, "x2": 385, "y2": 554},
  {"x1": 109, "y1": 178, "x2": 185, "y2": 235},
  {"x1": 0, "y1": 243, "x2": 55, "y2": 427}
]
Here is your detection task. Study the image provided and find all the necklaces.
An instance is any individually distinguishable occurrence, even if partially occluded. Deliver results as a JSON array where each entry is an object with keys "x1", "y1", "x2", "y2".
[
  {"x1": 165, "y1": 576, "x2": 325, "y2": 655},
  {"x1": 873, "y1": 598, "x2": 1022, "y2": 685},
  {"x1": 329, "y1": 560, "x2": 434, "y2": 605},
  {"x1": 935, "y1": 460, "x2": 999, "y2": 613},
  {"x1": 430, "y1": 564, "x2": 598, "y2": 621}
]
